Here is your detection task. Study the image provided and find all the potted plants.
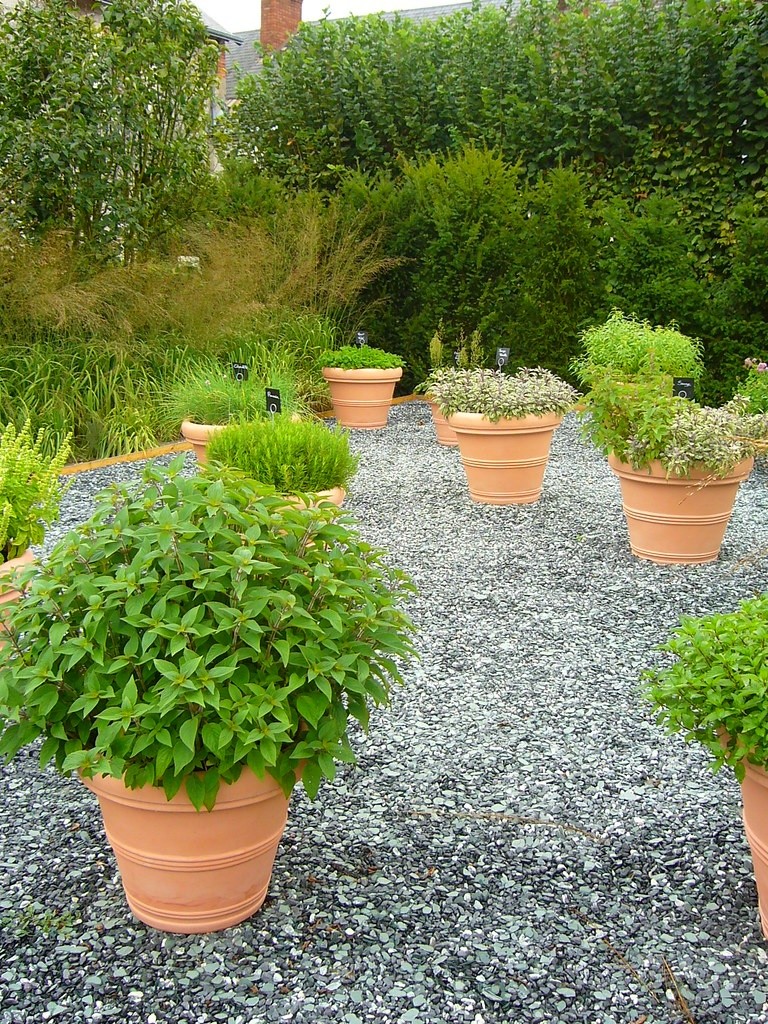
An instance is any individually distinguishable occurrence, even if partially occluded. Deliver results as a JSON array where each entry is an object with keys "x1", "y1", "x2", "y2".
[{"x1": 0, "y1": 311, "x2": 768, "y2": 943}]
